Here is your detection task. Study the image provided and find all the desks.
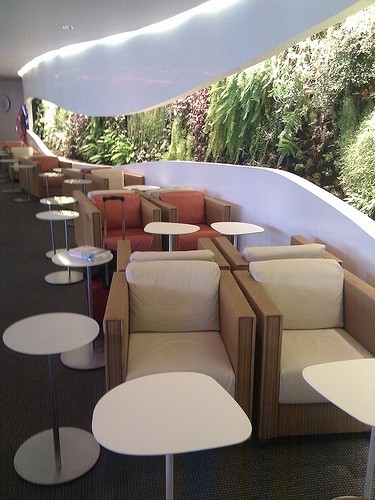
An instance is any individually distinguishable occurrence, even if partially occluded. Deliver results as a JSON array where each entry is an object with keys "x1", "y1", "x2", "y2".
[
  {"x1": 210, "y1": 221, "x2": 264, "y2": 250},
  {"x1": 36, "y1": 210, "x2": 84, "y2": 284},
  {"x1": 64, "y1": 179, "x2": 92, "y2": 195},
  {"x1": 0, "y1": 152, "x2": 9, "y2": 178},
  {"x1": 302, "y1": 358, "x2": 375, "y2": 500},
  {"x1": 38, "y1": 172, "x2": 65, "y2": 197},
  {"x1": 53, "y1": 168, "x2": 81, "y2": 173},
  {"x1": 92, "y1": 372, "x2": 253, "y2": 500},
  {"x1": 144, "y1": 222, "x2": 200, "y2": 251},
  {"x1": 52, "y1": 251, "x2": 113, "y2": 370},
  {"x1": 122, "y1": 184, "x2": 160, "y2": 193},
  {"x1": 3, "y1": 312, "x2": 100, "y2": 484},
  {"x1": 40, "y1": 196, "x2": 77, "y2": 259},
  {"x1": 0, "y1": 159, "x2": 22, "y2": 193},
  {"x1": 11, "y1": 164, "x2": 36, "y2": 203}
]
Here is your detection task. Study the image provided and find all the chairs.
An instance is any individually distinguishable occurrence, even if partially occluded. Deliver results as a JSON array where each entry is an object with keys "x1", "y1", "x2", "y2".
[{"x1": 0, "y1": 141, "x2": 375, "y2": 441}]
